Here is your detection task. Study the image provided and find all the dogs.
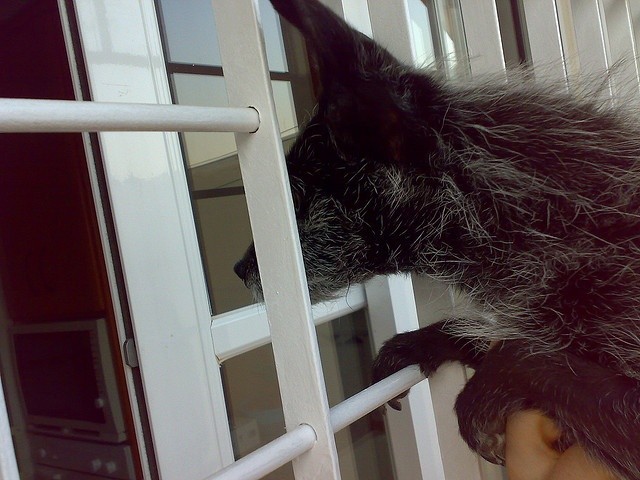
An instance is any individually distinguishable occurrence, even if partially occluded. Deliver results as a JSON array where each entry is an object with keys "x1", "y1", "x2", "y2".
[{"x1": 230, "y1": 0, "x2": 640, "y2": 479}]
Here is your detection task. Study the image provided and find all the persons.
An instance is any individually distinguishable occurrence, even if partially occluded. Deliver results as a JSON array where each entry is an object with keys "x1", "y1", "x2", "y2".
[{"x1": 504, "y1": 407, "x2": 627, "y2": 479}]
